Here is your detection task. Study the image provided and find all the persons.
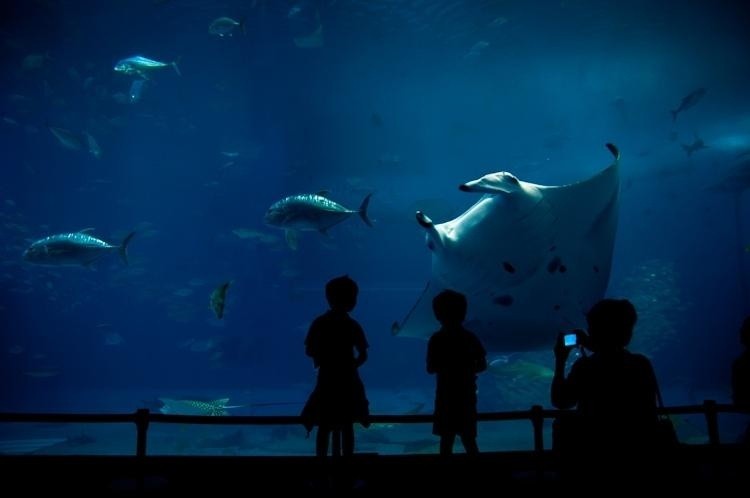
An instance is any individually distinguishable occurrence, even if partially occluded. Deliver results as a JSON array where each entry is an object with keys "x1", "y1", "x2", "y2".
[
  {"x1": 731, "y1": 316, "x2": 750, "y2": 498},
  {"x1": 301, "y1": 277, "x2": 370, "y2": 456},
  {"x1": 551, "y1": 299, "x2": 663, "y2": 498},
  {"x1": 427, "y1": 290, "x2": 486, "y2": 454}
]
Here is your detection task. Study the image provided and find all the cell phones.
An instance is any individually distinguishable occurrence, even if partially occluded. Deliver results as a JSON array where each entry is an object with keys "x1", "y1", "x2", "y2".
[{"x1": 562, "y1": 332, "x2": 580, "y2": 349}]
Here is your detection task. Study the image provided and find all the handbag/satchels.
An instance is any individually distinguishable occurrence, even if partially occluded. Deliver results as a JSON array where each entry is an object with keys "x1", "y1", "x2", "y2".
[{"x1": 659, "y1": 419, "x2": 678, "y2": 444}]
